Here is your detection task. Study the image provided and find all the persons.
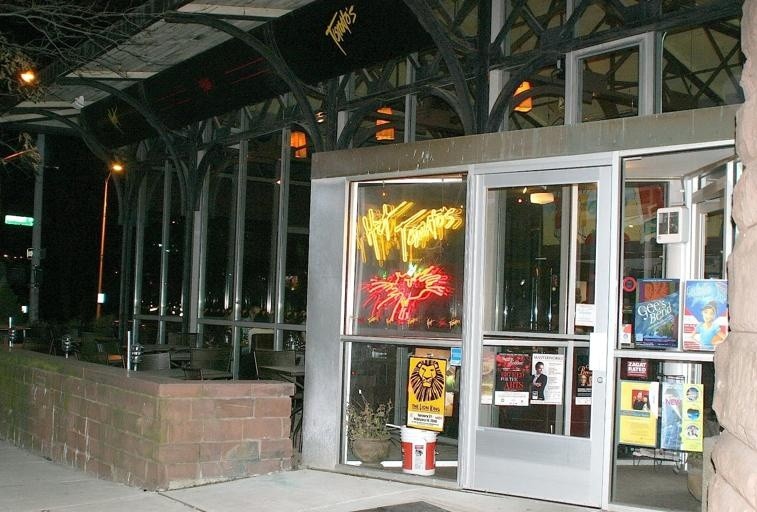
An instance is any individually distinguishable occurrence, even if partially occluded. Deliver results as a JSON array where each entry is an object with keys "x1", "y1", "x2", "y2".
[
  {"x1": 689, "y1": 301, "x2": 726, "y2": 350},
  {"x1": 531, "y1": 361, "x2": 547, "y2": 401},
  {"x1": 578, "y1": 374, "x2": 588, "y2": 385},
  {"x1": 632, "y1": 391, "x2": 649, "y2": 411}
]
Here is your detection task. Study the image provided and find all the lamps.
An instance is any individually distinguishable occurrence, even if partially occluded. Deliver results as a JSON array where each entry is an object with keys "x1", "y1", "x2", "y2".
[
  {"x1": 507, "y1": 17, "x2": 533, "y2": 113},
  {"x1": 289, "y1": 104, "x2": 307, "y2": 160},
  {"x1": 374, "y1": 108, "x2": 395, "y2": 141}
]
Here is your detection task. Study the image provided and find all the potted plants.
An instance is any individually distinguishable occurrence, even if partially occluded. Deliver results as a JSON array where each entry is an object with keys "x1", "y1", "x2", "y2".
[{"x1": 344, "y1": 392, "x2": 394, "y2": 469}]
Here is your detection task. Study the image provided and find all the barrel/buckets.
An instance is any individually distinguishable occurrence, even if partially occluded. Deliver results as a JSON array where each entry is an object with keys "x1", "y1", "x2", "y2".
[{"x1": 400, "y1": 425, "x2": 439, "y2": 477}]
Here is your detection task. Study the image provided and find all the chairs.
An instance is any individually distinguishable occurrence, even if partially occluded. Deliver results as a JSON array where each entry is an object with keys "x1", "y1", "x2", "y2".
[{"x1": 21, "y1": 322, "x2": 304, "y2": 433}]
